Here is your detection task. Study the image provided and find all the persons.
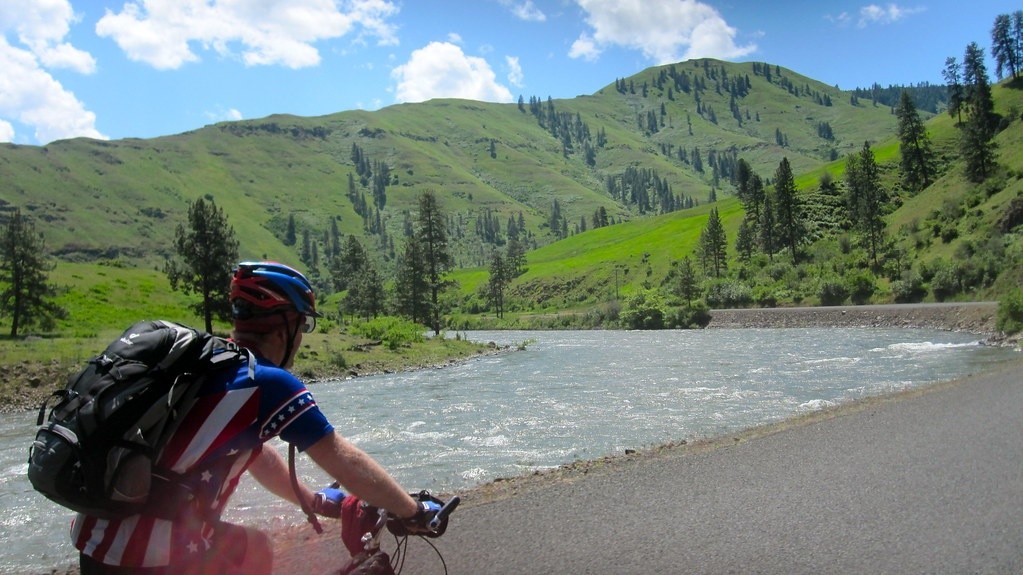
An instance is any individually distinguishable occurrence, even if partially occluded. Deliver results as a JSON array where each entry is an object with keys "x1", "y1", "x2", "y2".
[{"x1": 24, "y1": 258, "x2": 448, "y2": 574}]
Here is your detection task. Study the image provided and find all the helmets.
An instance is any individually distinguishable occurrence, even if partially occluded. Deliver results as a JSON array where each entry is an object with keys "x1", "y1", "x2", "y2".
[{"x1": 229, "y1": 261, "x2": 319, "y2": 332}]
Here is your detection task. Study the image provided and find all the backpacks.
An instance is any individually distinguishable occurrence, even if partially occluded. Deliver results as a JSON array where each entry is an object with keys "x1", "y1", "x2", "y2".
[{"x1": 26, "y1": 318, "x2": 241, "y2": 518}]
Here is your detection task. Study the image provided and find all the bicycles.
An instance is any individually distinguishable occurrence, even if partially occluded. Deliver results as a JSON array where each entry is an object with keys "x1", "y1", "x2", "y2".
[{"x1": 292, "y1": 478, "x2": 461, "y2": 574}]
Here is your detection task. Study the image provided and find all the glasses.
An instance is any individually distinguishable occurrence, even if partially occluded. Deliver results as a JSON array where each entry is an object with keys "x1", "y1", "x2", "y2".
[{"x1": 305, "y1": 309, "x2": 316, "y2": 331}]
